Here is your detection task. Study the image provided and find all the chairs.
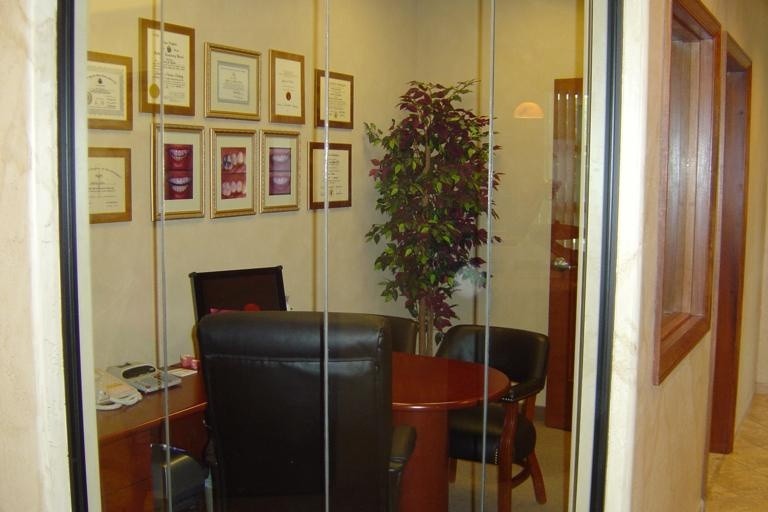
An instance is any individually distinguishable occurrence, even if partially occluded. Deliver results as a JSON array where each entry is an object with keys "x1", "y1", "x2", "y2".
[
  {"x1": 187, "y1": 266, "x2": 287, "y2": 316},
  {"x1": 197, "y1": 312, "x2": 417, "y2": 511},
  {"x1": 436, "y1": 324, "x2": 547, "y2": 511},
  {"x1": 384, "y1": 316, "x2": 418, "y2": 355}
]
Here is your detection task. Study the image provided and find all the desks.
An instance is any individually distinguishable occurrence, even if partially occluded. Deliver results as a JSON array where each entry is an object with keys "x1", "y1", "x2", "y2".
[{"x1": 387, "y1": 353, "x2": 512, "y2": 511}]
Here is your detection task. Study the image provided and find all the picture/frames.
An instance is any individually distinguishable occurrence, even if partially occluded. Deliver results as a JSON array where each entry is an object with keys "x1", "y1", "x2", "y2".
[
  {"x1": 259, "y1": 128, "x2": 303, "y2": 215},
  {"x1": 209, "y1": 124, "x2": 258, "y2": 221},
  {"x1": 86, "y1": 146, "x2": 132, "y2": 226},
  {"x1": 148, "y1": 122, "x2": 208, "y2": 223},
  {"x1": 85, "y1": 51, "x2": 134, "y2": 132}
]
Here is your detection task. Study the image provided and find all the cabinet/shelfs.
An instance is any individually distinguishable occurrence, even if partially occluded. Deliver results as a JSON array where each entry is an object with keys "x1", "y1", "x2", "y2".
[{"x1": 98, "y1": 358, "x2": 207, "y2": 512}]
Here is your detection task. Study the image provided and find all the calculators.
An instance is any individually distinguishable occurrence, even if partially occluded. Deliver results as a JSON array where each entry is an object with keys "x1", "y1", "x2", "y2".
[{"x1": 121, "y1": 364, "x2": 184, "y2": 393}]
